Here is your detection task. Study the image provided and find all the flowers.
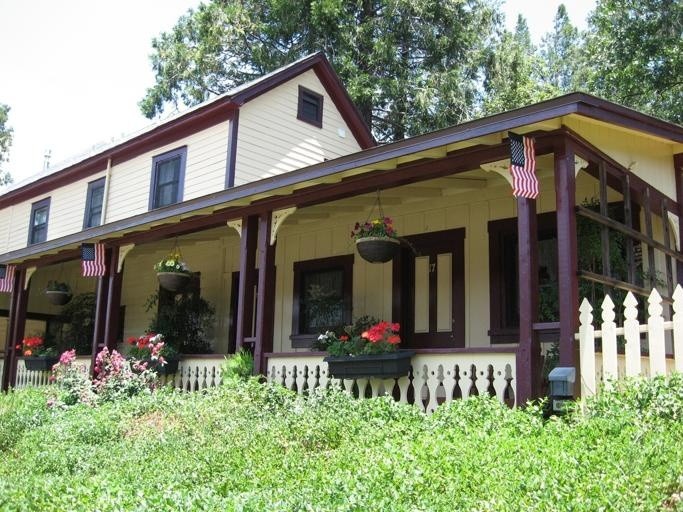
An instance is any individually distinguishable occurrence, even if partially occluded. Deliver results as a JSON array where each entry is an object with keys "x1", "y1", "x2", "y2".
[
  {"x1": 351, "y1": 216, "x2": 398, "y2": 241},
  {"x1": 16, "y1": 336, "x2": 61, "y2": 358},
  {"x1": 152, "y1": 254, "x2": 193, "y2": 277},
  {"x1": 317, "y1": 315, "x2": 401, "y2": 355},
  {"x1": 125, "y1": 330, "x2": 179, "y2": 361}
]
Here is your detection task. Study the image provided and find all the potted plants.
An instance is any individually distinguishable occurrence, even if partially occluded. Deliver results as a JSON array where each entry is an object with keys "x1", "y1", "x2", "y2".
[{"x1": 42, "y1": 280, "x2": 73, "y2": 307}]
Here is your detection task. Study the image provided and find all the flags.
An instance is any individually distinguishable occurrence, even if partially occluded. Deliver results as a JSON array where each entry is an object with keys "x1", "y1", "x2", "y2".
[
  {"x1": 506, "y1": 129, "x2": 540, "y2": 200},
  {"x1": 0, "y1": 264, "x2": 14, "y2": 294},
  {"x1": 79, "y1": 242, "x2": 107, "y2": 278}
]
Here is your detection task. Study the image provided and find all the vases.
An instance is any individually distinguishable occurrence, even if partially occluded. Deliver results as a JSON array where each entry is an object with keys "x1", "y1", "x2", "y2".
[
  {"x1": 130, "y1": 359, "x2": 178, "y2": 375},
  {"x1": 323, "y1": 349, "x2": 416, "y2": 378},
  {"x1": 156, "y1": 272, "x2": 190, "y2": 291},
  {"x1": 354, "y1": 236, "x2": 400, "y2": 264},
  {"x1": 23, "y1": 355, "x2": 59, "y2": 370}
]
